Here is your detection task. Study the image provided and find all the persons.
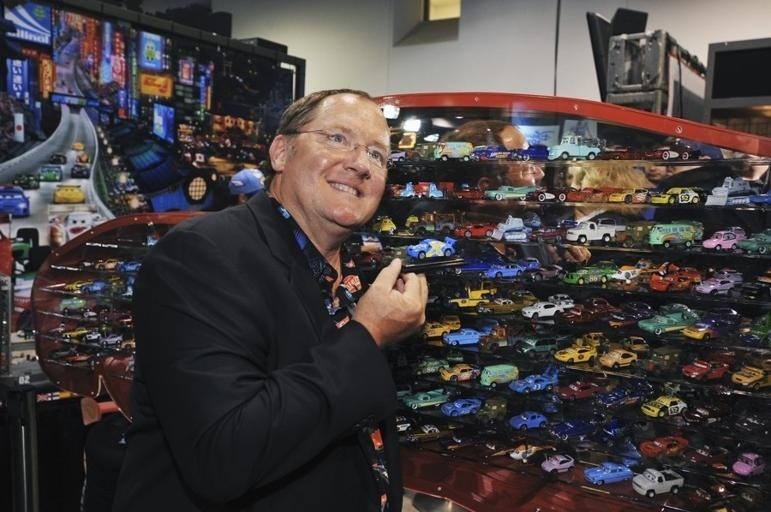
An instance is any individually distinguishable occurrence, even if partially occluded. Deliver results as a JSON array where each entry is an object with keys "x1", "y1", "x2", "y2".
[{"x1": 124, "y1": 89, "x2": 430, "y2": 512}]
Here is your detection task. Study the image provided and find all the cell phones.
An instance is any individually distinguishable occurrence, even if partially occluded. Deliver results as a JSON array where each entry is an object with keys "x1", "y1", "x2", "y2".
[{"x1": 391, "y1": 255, "x2": 465, "y2": 273}]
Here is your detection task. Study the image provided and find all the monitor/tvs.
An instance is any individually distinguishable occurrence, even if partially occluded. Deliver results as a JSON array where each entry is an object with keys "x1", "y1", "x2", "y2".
[
  {"x1": 587, "y1": 7, "x2": 648, "y2": 103},
  {"x1": 704, "y1": 37, "x2": 771, "y2": 108}
]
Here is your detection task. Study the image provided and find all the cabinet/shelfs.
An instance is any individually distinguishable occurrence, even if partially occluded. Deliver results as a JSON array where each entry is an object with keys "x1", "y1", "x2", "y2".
[
  {"x1": 345, "y1": 154, "x2": 771, "y2": 511},
  {"x1": 36, "y1": 216, "x2": 208, "y2": 422},
  {"x1": 1, "y1": 0, "x2": 303, "y2": 392}
]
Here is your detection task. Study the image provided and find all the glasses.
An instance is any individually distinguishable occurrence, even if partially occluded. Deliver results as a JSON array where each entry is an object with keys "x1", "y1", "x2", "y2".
[{"x1": 280, "y1": 128, "x2": 389, "y2": 169}]
